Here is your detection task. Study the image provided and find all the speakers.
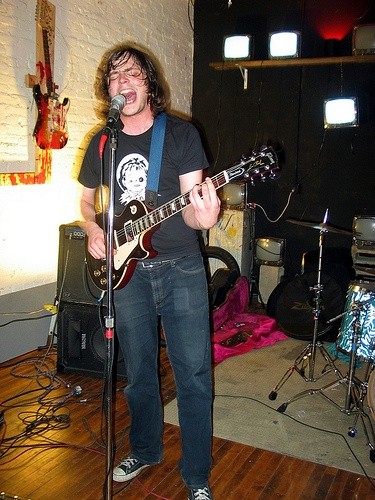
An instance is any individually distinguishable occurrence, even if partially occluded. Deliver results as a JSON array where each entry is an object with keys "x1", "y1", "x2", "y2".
[
  {"x1": 55, "y1": 220, "x2": 108, "y2": 305},
  {"x1": 208, "y1": 206, "x2": 255, "y2": 289},
  {"x1": 56, "y1": 299, "x2": 129, "y2": 383}
]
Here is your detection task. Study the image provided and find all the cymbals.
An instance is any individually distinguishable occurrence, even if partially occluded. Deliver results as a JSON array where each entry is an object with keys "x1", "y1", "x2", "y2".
[{"x1": 286, "y1": 219, "x2": 362, "y2": 236}]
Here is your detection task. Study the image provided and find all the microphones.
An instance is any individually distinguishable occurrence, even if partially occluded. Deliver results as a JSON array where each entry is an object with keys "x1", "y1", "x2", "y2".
[{"x1": 102, "y1": 93, "x2": 128, "y2": 139}]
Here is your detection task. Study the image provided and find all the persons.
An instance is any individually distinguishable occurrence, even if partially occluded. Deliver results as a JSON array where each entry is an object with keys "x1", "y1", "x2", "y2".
[{"x1": 77, "y1": 47, "x2": 221, "y2": 500}]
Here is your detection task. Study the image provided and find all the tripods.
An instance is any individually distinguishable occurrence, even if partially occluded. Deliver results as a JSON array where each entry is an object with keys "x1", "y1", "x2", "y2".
[{"x1": 266, "y1": 230, "x2": 375, "y2": 463}]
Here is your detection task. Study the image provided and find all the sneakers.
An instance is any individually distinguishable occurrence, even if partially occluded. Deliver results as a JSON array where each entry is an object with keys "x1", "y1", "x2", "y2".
[
  {"x1": 188, "y1": 479, "x2": 212, "y2": 500},
  {"x1": 112, "y1": 455, "x2": 151, "y2": 483}
]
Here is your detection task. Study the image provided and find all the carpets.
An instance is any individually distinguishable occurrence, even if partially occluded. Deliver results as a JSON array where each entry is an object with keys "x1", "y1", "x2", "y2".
[{"x1": 162, "y1": 337, "x2": 375, "y2": 478}]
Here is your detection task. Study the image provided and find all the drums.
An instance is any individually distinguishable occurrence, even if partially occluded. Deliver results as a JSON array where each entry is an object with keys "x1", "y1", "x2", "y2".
[{"x1": 335, "y1": 280, "x2": 375, "y2": 363}]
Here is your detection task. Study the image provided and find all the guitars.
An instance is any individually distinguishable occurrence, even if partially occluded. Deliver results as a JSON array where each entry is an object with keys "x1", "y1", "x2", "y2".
[
  {"x1": 86, "y1": 145, "x2": 280, "y2": 292},
  {"x1": 34, "y1": 0, "x2": 70, "y2": 149}
]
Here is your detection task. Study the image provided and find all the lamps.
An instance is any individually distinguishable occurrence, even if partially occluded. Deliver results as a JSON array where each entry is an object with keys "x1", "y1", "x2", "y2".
[
  {"x1": 351, "y1": 21, "x2": 375, "y2": 56},
  {"x1": 254, "y1": 236, "x2": 287, "y2": 266},
  {"x1": 266, "y1": 30, "x2": 300, "y2": 60},
  {"x1": 217, "y1": 181, "x2": 247, "y2": 210},
  {"x1": 221, "y1": 33, "x2": 253, "y2": 60},
  {"x1": 352, "y1": 215, "x2": 375, "y2": 246},
  {"x1": 323, "y1": 95, "x2": 359, "y2": 128}
]
